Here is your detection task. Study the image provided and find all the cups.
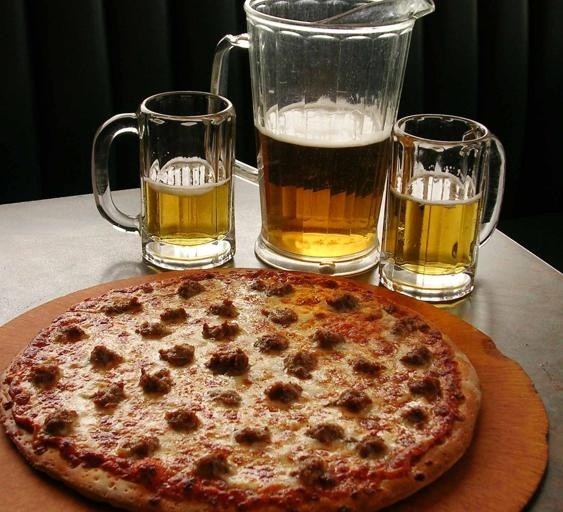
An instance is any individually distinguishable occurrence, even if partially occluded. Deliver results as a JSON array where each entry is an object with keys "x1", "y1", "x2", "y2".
[
  {"x1": 90, "y1": 93, "x2": 239, "y2": 279},
  {"x1": 384, "y1": 115, "x2": 506, "y2": 306},
  {"x1": 208, "y1": 0, "x2": 435, "y2": 277}
]
